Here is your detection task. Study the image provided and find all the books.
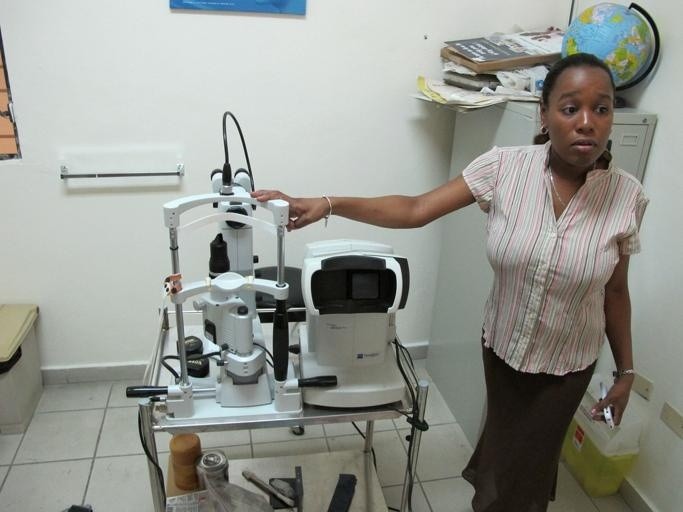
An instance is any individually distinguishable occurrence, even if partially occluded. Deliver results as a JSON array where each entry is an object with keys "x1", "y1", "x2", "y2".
[{"x1": 410, "y1": 27, "x2": 567, "y2": 114}]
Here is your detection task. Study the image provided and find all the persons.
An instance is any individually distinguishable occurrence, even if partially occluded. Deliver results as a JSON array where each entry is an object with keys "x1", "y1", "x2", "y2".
[{"x1": 250, "y1": 54, "x2": 649, "y2": 512}]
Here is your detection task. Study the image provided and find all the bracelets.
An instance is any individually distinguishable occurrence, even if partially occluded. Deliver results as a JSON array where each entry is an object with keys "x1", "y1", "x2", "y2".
[
  {"x1": 612, "y1": 369, "x2": 636, "y2": 378},
  {"x1": 322, "y1": 194, "x2": 332, "y2": 228}
]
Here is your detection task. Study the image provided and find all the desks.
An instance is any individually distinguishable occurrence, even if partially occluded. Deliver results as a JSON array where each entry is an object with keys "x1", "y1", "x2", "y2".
[{"x1": 133, "y1": 304, "x2": 430, "y2": 511}]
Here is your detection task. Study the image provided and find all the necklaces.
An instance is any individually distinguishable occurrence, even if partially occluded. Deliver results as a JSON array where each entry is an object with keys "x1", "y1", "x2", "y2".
[{"x1": 547, "y1": 164, "x2": 597, "y2": 208}]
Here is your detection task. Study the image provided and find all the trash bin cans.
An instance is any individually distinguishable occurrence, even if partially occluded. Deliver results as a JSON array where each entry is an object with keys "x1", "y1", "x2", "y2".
[{"x1": 0, "y1": 303, "x2": 44, "y2": 436}]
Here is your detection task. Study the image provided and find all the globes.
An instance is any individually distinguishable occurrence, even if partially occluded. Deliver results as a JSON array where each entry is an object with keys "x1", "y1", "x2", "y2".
[{"x1": 560, "y1": 2, "x2": 660, "y2": 107}]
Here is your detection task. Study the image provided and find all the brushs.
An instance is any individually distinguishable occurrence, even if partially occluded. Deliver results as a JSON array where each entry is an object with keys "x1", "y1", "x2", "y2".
[{"x1": 242, "y1": 468, "x2": 295, "y2": 507}]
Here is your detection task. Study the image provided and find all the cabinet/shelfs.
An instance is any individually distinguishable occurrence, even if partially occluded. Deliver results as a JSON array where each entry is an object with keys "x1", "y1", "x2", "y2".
[{"x1": 420, "y1": 95, "x2": 658, "y2": 452}]
